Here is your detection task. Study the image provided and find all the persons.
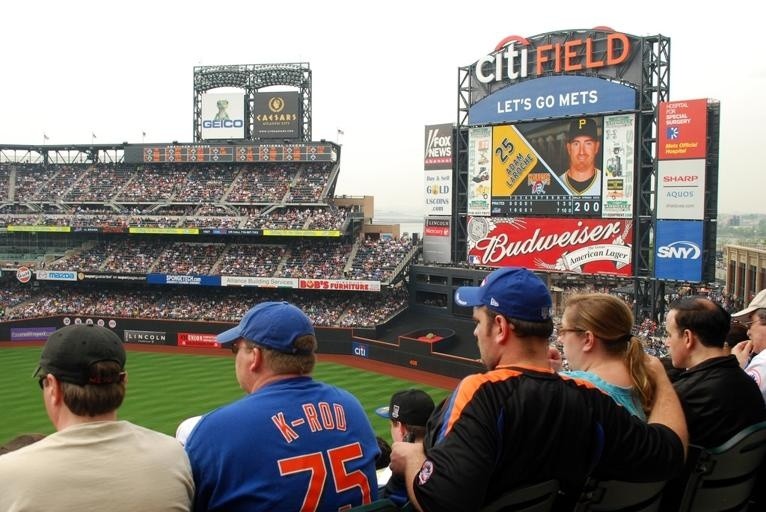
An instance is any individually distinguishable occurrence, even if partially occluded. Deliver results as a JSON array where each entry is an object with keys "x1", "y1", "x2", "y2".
[
  {"x1": 377, "y1": 266, "x2": 765, "y2": 512},
  {"x1": 1, "y1": 164, "x2": 355, "y2": 230},
  {"x1": 558, "y1": 119, "x2": 602, "y2": 199},
  {"x1": 0, "y1": 302, "x2": 394, "y2": 511},
  {"x1": 1, "y1": 227, "x2": 421, "y2": 282},
  {"x1": 1, "y1": 271, "x2": 410, "y2": 328}
]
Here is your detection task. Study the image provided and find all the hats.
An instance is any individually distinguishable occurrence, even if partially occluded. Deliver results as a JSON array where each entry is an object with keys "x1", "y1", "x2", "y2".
[
  {"x1": 731, "y1": 289, "x2": 766, "y2": 318},
  {"x1": 214, "y1": 302, "x2": 318, "y2": 356},
  {"x1": 31, "y1": 324, "x2": 128, "y2": 388},
  {"x1": 566, "y1": 118, "x2": 598, "y2": 142},
  {"x1": 454, "y1": 268, "x2": 552, "y2": 324},
  {"x1": 375, "y1": 389, "x2": 435, "y2": 428}
]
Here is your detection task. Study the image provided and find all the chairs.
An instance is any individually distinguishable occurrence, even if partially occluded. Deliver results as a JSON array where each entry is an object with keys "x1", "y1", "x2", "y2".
[
  {"x1": 332, "y1": 417, "x2": 765, "y2": 512},
  {"x1": 0, "y1": 282, "x2": 415, "y2": 330},
  {"x1": 1, "y1": 205, "x2": 350, "y2": 231},
  {"x1": 2, "y1": 232, "x2": 415, "y2": 282},
  {"x1": 1, "y1": 161, "x2": 336, "y2": 205}
]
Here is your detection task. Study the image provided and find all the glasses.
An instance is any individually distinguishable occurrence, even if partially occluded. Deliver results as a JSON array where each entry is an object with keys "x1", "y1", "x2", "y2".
[
  {"x1": 229, "y1": 343, "x2": 248, "y2": 355},
  {"x1": 744, "y1": 319, "x2": 764, "y2": 329},
  {"x1": 37, "y1": 373, "x2": 48, "y2": 388},
  {"x1": 556, "y1": 327, "x2": 581, "y2": 335}
]
